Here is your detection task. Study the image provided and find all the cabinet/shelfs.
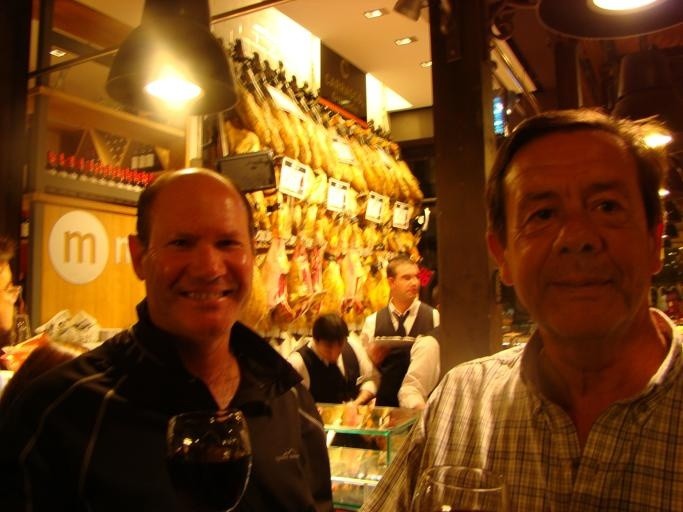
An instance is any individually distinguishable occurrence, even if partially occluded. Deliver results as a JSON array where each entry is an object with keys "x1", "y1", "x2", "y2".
[{"x1": 314, "y1": 405, "x2": 421, "y2": 510}]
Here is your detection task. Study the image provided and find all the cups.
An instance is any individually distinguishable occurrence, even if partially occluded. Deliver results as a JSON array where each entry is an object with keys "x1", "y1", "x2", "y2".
[
  {"x1": 162, "y1": 407, "x2": 257, "y2": 512},
  {"x1": 410, "y1": 464, "x2": 512, "y2": 511}
]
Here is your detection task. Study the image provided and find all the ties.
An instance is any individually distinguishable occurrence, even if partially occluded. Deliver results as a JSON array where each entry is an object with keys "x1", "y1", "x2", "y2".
[
  {"x1": 393, "y1": 312, "x2": 409, "y2": 338},
  {"x1": 327, "y1": 362, "x2": 343, "y2": 381}
]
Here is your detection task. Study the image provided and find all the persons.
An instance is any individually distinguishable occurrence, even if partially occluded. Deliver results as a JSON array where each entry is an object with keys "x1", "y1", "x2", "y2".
[
  {"x1": 2, "y1": 337, "x2": 91, "y2": 400},
  {"x1": 354, "y1": 108, "x2": 683, "y2": 511},
  {"x1": 1, "y1": 167, "x2": 334, "y2": 512},
  {"x1": 352, "y1": 255, "x2": 440, "y2": 372},
  {"x1": 287, "y1": 314, "x2": 382, "y2": 405},
  {"x1": 0, "y1": 234, "x2": 25, "y2": 344},
  {"x1": 370, "y1": 325, "x2": 442, "y2": 408}
]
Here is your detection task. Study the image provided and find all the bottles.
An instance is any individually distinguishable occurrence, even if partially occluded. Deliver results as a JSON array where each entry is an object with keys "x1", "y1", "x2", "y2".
[{"x1": 42, "y1": 151, "x2": 161, "y2": 209}]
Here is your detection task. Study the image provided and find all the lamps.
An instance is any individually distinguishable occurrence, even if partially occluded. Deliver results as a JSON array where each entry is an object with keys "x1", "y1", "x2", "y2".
[
  {"x1": 536, "y1": 0, "x2": 683, "y2": 41},
  {"x1": 107, "y1": 0, "x2": 239, "y2": 118}
]
